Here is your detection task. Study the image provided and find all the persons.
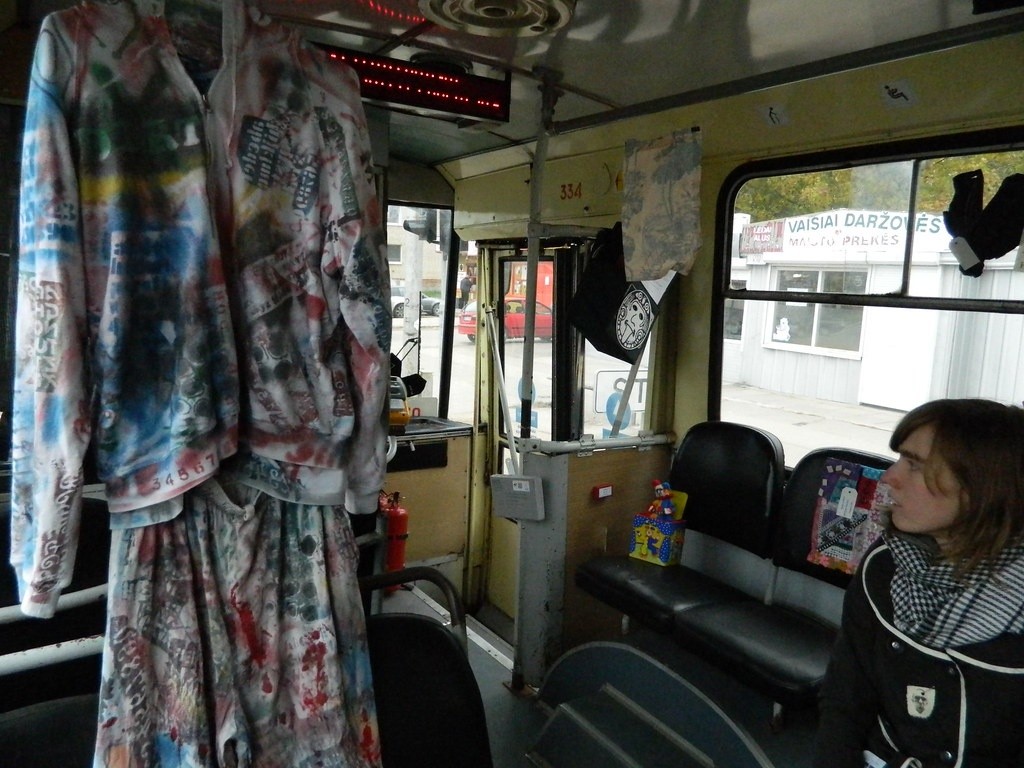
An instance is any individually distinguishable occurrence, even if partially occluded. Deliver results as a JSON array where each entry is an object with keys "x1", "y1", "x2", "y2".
[
  {"x1": 647, "y1": 480, "x2": 676, "y2": 521},
  {"x1": 816, "y1": 398, "x2": 1024, "y2": 768},
  {"x1": 460, "y1": 275, "x2": 472, "y2": 307}
]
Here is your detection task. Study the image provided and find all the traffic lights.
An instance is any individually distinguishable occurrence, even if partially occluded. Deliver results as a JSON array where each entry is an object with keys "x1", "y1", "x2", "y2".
[{"x1": 403, "y1": 206, "x2": 438, "y2": 241}]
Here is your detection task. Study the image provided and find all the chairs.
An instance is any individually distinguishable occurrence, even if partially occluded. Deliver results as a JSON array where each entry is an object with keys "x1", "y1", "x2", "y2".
[
  {"x1": 507, "y1": 305, "x2": 511, "y2": 313},
  {"x1": 671, "y1": 446, "x2": 895, "y2": 735},
  {"x1": 515, "y1": 307, "x2": 523, "y2": 313},
  {"x1": 573, "y1": 418, "x2": 785, "y2": 636}
]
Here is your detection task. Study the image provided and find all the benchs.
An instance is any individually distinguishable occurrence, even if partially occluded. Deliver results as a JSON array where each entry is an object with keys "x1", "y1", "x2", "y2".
[{"x1": 0, "y1": 564, "x2": 494, "y2": 768}]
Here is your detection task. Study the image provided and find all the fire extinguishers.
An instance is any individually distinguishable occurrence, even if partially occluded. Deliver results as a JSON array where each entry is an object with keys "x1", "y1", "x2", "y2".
[{"x1": 380, "y1": 488, "x2": 409, "y2": 593}]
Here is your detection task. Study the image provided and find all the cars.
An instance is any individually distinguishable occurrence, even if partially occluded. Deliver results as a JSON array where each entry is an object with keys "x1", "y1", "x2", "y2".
[
  {"x1": 390, "y1": 286, "x2": 407, "y2": 318},
  {"x1": 420, "y1": 291, "x2": 441, "y2": 317},
  {"x1": 458, "y1": 297, "x2": 553, "y2": 343}
]
